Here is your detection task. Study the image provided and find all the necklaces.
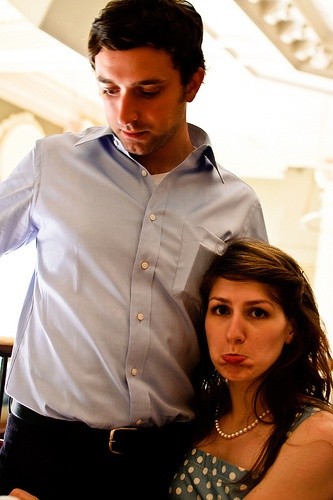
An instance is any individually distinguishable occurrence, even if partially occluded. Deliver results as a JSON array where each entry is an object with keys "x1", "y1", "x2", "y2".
[{"x1": 214, "y1": 410, "x2": 269, "y2": 440}]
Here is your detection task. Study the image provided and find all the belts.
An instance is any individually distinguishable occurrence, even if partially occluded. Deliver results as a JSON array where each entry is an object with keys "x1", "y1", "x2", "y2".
[{"x1": 8, "y1": 404, "x2": 192, "y2": 456}]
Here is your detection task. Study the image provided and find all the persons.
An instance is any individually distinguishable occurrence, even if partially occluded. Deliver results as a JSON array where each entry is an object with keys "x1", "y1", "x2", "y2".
[
  {"x1": 0, "y1": 239, "x2": 333, "y2": 500},
  {"x1": 0, "y1": 0, "x2": 269, "y2": 500}
]
